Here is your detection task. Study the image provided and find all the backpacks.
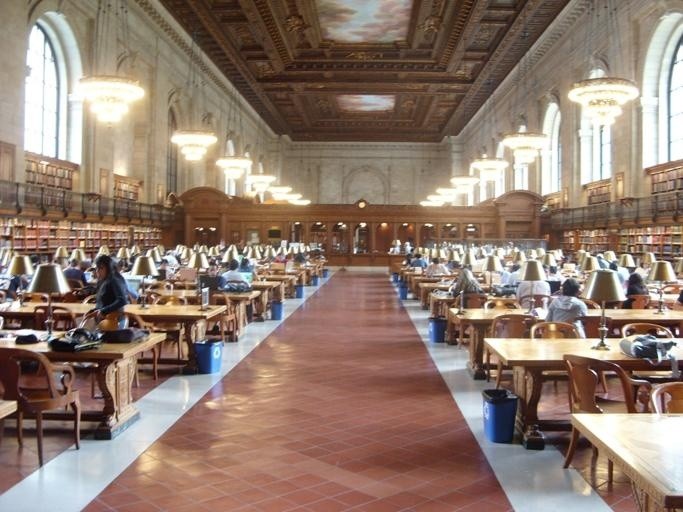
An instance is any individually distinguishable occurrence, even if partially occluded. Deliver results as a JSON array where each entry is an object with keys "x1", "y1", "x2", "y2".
[{"x1": 620, "y1": 334, "x2": 678, "y2": 378}]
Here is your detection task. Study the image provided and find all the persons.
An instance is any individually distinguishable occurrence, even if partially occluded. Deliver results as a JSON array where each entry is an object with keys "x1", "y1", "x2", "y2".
[
  {"x1": 0, "y1": 250, "x2": 181, "y2": 398},
  {"x1": 207, "y1": 250, "x2": 325, "y2": 341},
  {"x1": 390, "y1": 238, "x2": 682, "y2": 343}
]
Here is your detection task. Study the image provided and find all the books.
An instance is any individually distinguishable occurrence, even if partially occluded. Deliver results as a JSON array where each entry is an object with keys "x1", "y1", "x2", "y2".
[
  {"x1": 1, "y1": 157, "x2": 162, "y2": 254},
  {"x1": 560, "y1": 167, "x2": 683, "y2": 263}
]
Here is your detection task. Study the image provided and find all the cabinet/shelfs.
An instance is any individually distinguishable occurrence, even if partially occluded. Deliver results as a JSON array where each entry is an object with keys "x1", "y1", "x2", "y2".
[
  {"x1": 564, "y1": 216, "x2": 683, "y2": 273},
  {"x1": 1, "y1": 214, "x2": 164, "y2": 262}
]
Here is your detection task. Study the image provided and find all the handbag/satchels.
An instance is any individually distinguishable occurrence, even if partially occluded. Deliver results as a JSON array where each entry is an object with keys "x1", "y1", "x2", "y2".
[
  {"x1": 101, "y1": 326, "x2": 150, "y2": 342},
  {"x1": 50, "y1": 328, "x2": 102, "y2": 352}
]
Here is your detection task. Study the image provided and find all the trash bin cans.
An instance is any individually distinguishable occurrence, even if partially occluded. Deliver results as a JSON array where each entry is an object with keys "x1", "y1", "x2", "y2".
[
  {"x1": 271, "y1": 301, "x2": 283, "y2": 320},
  {"x1": 295, "y1": 285, "x2": 303, "y2": 298},
  {"x1": 393, "y1": 272, "x2": 398, "y2": 282},
  {"x1": 398, "y1": 281, "x2": 408, "y2": 299},
  {"x1": 482, "y1": 389, "x2": 518, "y2": 443},
  {"x1": 312, "y1": 275, "x2": 320, "y2": 285},
  {"x1": 428, "y1": 318, "x2": 446, "y2": 343},
  {"x1": 194, "y1": 338, "x2": 223, "y2": 373},
  {"x1": 323, "y1": 269, "x2": 329, "y2": 277}
]
